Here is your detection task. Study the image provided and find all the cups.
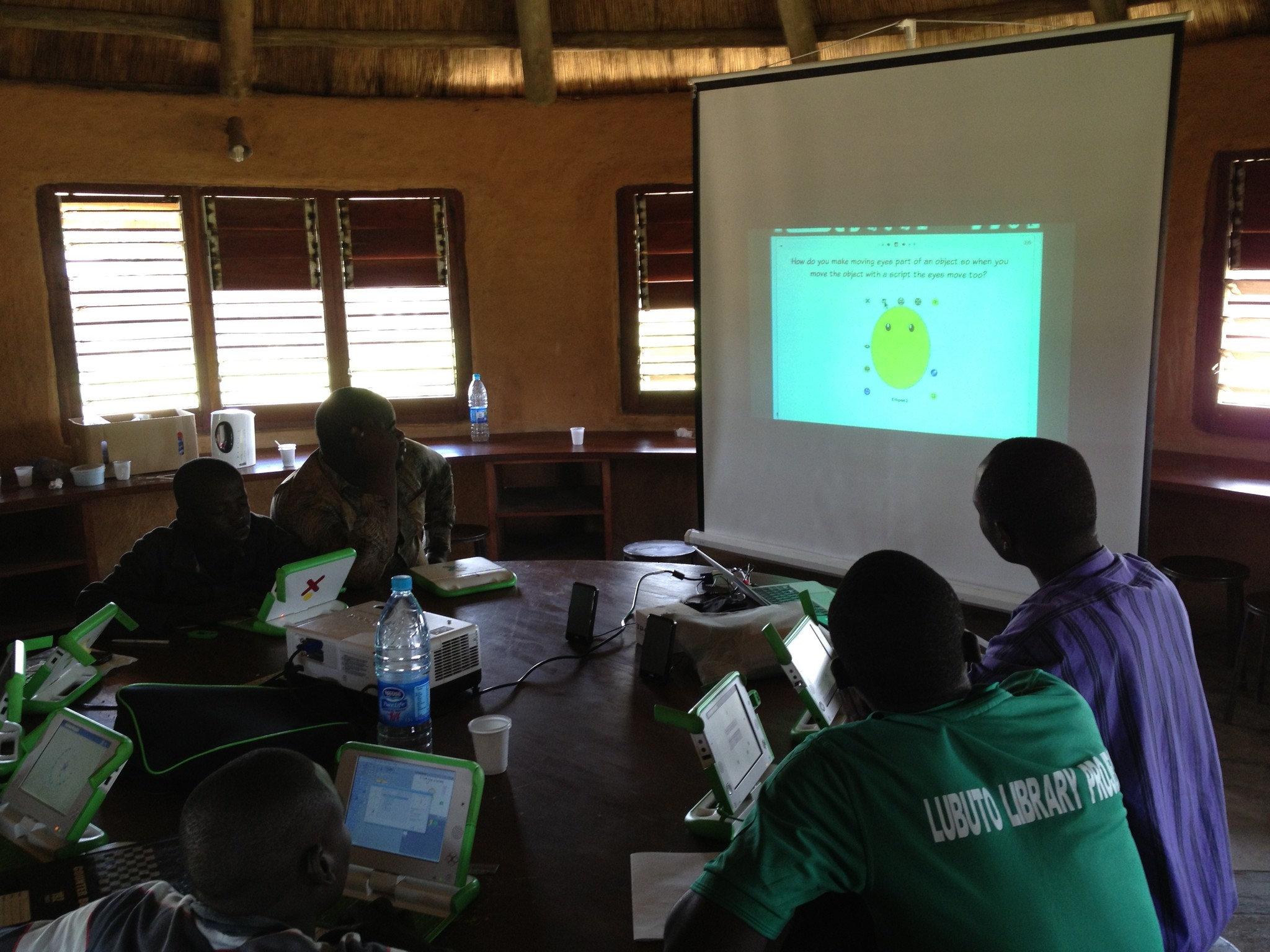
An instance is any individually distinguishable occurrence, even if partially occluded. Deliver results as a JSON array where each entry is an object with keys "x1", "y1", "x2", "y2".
[
  {"x1": 570, "y1": 427, "x2": 585, "y2": 444},
  {"x1": 14, "y1": 466, "x2": 33, "y2": 487},
  {"x1": 278, "y1": 444, "x2": 297, "y2": 467},
  {"x1": 113, "y1": 460, "x2": 132, "y2": 480},
  {"x1": 468, "y1": 715, "x2": 511, "y2": 774}
]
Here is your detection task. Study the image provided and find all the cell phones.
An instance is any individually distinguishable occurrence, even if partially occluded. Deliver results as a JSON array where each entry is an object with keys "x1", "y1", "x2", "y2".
[{"x1": 566, "y1": 581, "x2": 598, "y2": 650}]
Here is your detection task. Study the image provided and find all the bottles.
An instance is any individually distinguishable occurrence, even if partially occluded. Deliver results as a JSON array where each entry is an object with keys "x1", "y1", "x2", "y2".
[
  {"x1": 468, "y1": 374, "x2": 489, "y2": 442},
  {"x1": 374, "y1": 575, "x2": 434, "y2": 754}
]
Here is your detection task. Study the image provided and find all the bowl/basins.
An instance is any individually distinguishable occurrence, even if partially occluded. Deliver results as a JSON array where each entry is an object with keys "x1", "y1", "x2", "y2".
[{"x1": 70, "y1": 462, "x2": 105, "y2": 485}]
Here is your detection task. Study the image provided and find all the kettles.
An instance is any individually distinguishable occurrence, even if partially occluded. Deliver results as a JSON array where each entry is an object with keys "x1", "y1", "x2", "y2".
[{"x1": 210, "y1": 409, "x2": 257, "y2": 469}]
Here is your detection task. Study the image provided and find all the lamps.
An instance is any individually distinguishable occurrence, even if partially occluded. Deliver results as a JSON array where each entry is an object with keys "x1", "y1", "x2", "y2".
[{"x1": 227, "y1": 115, "x2": 253, "y2": 163}]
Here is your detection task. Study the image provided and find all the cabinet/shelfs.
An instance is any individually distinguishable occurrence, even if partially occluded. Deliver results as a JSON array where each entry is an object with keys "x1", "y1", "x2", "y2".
[
  {"x1": 0, "y1": 430, "x2": 697, "y2": 643},
  {"x1": 1147, "y1": 442, "x2": 1270, "y2": 602}
]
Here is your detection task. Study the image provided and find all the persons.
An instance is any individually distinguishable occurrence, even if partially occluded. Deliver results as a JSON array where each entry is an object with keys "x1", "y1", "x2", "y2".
[
  {"x1": 661, "y1": 548, "x2": 1169, "y2": 952},
  {"x1": 269, "y1": 386, "x2": 457, "y2": 598},
  {"x1": 962, "y1": 436, "x2": 1238, "y2": 952},
  {"x1": 66, "y1": 457, "x2": 300, "y2": 652},
  {"x1": 4, "y1": 749, "x2": 417, "y2": 951}
]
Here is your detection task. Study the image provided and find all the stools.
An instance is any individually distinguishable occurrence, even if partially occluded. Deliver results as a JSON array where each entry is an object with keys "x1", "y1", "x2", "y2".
[
  {"x1": 451, "y1": 524, "x2": 490, "y2": 559},
  {"x1": 620, "y1": 539, "x2": 697, "y2": 564},
  {"x1": 1155, "y1": 555, "x2": 1270, "y2": 726}
]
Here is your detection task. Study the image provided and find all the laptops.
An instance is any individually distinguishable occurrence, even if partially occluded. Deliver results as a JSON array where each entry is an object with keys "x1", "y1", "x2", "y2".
[
  {"x1": 653, "y1": 545, "x2": 860, "y2": 840},
  {"x1": 0, "y1": 602, "x2": 137, "y2": 875},
  {"x1": 407, "y1": 556, "x2": 517, "y2": 598},
  {"x1": 215, "y1": 547, "x2": 356, "y2": 635},
  {"x1": 319, "y1": 742, "x2": 485, "y2": 952}
]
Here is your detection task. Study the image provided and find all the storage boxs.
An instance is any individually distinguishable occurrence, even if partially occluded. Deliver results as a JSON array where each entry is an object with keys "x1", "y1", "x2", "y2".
[{"x1": 65, "y1": 407, "x2": 200, "y2": 478}]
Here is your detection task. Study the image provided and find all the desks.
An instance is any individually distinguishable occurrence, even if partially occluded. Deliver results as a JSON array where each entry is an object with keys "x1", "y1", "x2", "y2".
[{"x1": 71, "y1": 559, "x2": 989, "y2": 952}]
